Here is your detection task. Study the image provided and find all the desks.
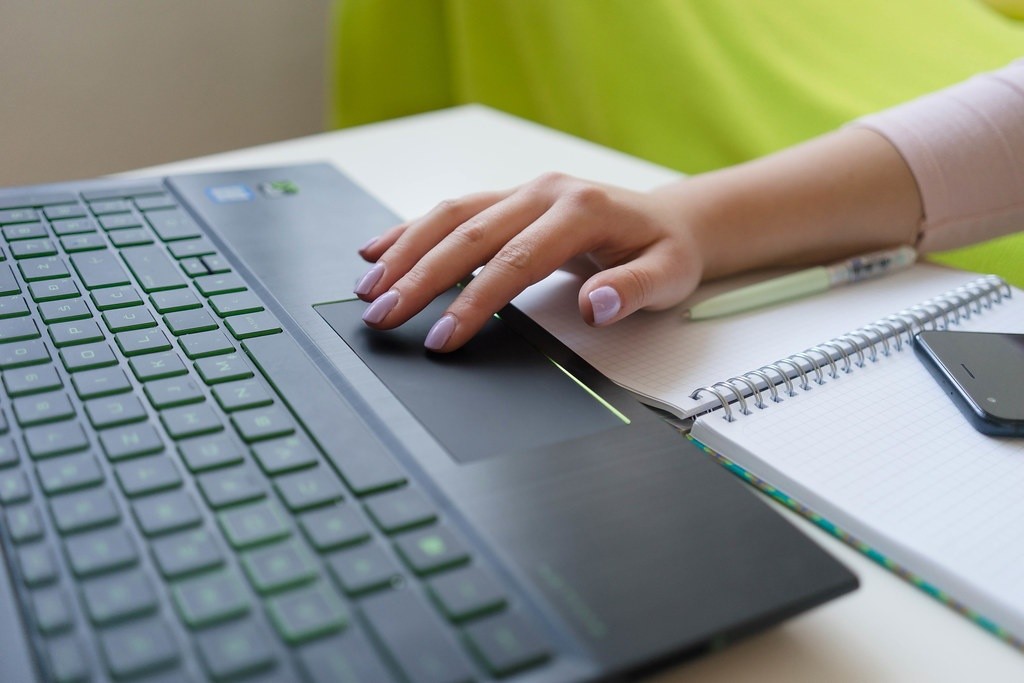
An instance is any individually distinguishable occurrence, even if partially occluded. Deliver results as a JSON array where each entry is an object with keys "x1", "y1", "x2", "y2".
[{"x1": 109, "y1": 105, "x2": 1024, "y2": 683}]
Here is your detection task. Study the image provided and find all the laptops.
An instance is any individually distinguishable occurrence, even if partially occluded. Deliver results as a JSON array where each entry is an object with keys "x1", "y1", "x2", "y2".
[{"x1": 0, "y1": 160, "x2": 861, "y2": 683}]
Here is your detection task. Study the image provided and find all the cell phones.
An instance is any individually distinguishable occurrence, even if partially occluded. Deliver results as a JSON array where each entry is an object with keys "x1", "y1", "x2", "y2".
[{"x1": 915, "y1": 329, "x2": 1024, "y2": 438}]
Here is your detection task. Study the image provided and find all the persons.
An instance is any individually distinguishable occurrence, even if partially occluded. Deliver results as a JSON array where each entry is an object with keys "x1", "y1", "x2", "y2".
[{"x1": 354, "y1": 59, "x2": 1024, "y2": 351}]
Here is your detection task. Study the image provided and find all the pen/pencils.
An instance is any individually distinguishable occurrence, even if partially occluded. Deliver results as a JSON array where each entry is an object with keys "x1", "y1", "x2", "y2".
[{"x1": 682, "y1": 244, "x2": 917, "y2": 322}]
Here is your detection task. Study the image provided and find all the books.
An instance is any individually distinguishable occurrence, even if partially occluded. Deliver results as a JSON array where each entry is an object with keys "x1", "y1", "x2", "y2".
[{"x1": 467, "y1": 258, "x2": 1024, "y2": 649}]
